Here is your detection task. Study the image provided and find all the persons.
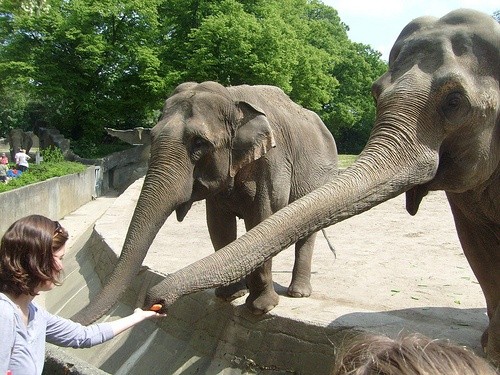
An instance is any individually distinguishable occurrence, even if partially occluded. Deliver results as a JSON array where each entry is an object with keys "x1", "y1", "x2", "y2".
[
  {"x1": 0, "y1": 149, "x2": 30, "y2": 184},
  {"x1": 0, "y1": 215, "x2": 166, "y2": 375}
]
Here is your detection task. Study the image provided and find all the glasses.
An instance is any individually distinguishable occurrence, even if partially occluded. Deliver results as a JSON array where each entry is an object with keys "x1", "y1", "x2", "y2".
[{"x1": 55, "y1": 255, "x2": 65, "y2": 263}]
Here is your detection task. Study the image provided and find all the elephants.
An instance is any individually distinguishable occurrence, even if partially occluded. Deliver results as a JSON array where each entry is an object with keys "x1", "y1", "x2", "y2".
[
  {"x1": 69, "y1": 81, "x2": 340, "y2": 325},
  {"x1": 142, "y1": 8, "x2": 499, "y2": 364}
]
